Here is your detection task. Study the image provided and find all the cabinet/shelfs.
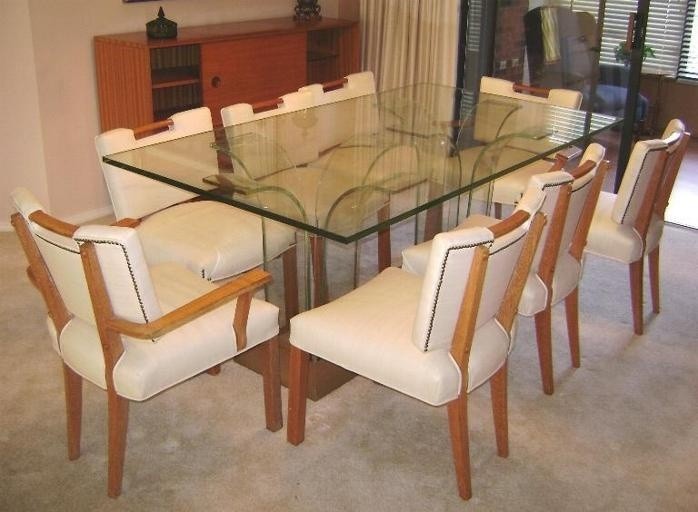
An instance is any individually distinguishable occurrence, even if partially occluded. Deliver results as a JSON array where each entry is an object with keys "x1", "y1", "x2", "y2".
[{"x1": 94, "y1": 14, "x2": 359, "y2": 147}]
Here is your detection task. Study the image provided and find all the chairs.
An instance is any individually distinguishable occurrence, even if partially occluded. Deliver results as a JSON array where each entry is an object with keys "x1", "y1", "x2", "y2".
[{"x1": 525, "y1": 6, "x2": 650, "y2": 132}]
